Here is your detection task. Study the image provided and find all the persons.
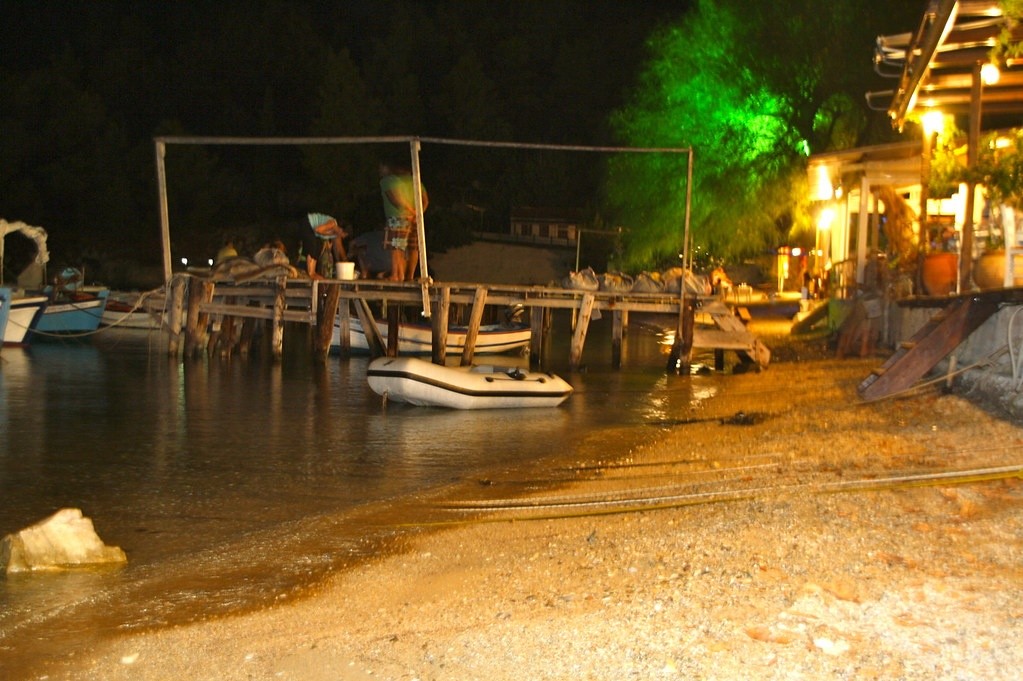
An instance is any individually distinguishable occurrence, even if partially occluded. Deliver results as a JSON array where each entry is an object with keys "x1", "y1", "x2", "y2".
[
  {"x1": 379, "y1": 158, "x2": 428, "y2": 283},
  {"x1": 710, "y1": 266, "x2": 732, "y2": 294},
  {"x1": 254, "y1": 239, "x2": 289, "y2": 267},
  {"x1": 213, "y1": 240, "x2": 238, "y2": 267}
]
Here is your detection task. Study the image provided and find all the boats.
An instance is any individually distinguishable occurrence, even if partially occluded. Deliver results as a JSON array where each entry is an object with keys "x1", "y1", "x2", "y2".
[
  {"x1": 0, "y1": 286, "x2": 188, "y2": 345},
  {"x1": 366, "y1": 357, "x2": 573, "y2": 411},
  {"x1": 329, "y1": 315, "x2": 532, "y2": 356}
]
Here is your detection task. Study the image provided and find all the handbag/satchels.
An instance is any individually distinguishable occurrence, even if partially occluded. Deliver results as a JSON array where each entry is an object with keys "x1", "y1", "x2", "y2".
[{"x1": 383, "y1": 218, "x2": 412, "y2": 250}]
